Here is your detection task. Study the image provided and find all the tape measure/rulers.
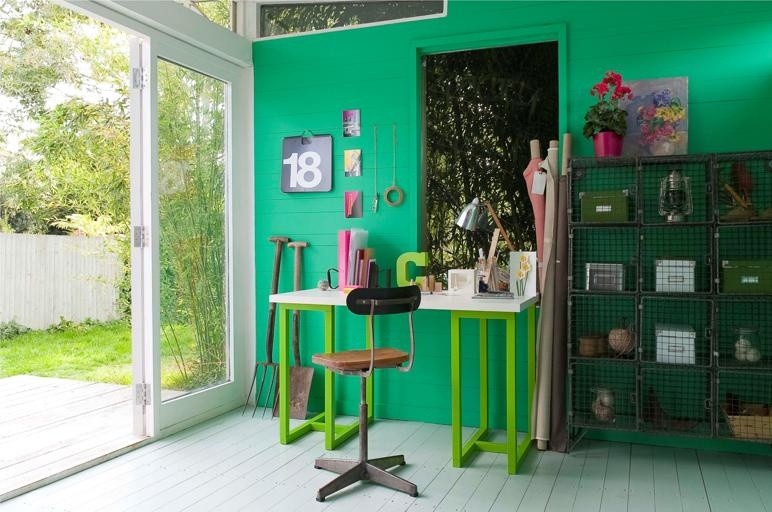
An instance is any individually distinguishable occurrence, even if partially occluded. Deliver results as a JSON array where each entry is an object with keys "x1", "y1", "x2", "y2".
[{"x1": 482, "y1": 228, "x2": 501, "y2": 284}]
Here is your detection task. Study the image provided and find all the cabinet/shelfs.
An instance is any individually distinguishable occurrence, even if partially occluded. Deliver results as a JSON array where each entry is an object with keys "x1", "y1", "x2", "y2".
[{"x1": 562, "y1": 148, "x2": 771, "y2": 455}]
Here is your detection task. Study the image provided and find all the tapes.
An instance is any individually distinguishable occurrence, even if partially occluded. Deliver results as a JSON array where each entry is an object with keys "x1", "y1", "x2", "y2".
[{"x1": 385, "y1": 187, "x2": 403, "y2": 206}]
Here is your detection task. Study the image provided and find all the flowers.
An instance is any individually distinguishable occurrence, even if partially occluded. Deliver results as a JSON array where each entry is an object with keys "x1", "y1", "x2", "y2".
[{"x1": 581, "y1": 71, "x2": 634, "y2": 141}]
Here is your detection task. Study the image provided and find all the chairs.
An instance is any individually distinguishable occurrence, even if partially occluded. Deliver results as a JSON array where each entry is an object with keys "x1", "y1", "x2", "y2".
[{"x1": 311, "y1": 287, "x2": 419, "y2": 502}]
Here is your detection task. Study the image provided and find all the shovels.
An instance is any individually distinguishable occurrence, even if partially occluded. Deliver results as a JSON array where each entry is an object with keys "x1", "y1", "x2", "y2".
[{"x1": 273, "y1": 242, "x2": 315, "y2": 420}]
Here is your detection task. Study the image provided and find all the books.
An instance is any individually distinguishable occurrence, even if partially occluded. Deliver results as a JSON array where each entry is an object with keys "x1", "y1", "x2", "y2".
[
  {"x1": 280, "y1": 135, "x2": 333, "y2": 193},
  {"x1": 338, "y1": 228, "x2": 379, "y2": 292}
]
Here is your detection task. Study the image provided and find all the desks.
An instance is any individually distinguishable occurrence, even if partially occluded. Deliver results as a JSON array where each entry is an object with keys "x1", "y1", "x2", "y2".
[{"x1": 268, "y1": 285, "x2": 541, "y2": 476}]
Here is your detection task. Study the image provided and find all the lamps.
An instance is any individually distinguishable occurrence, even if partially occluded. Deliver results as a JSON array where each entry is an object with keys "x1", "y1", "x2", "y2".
[{"x1": 456, "y1": 196, "x2": 516, "y2": 252}]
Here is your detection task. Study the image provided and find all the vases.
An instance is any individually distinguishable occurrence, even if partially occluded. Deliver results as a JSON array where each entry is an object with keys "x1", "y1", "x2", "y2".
[{"x1": 593, "y1": 130, "x2": 625, "y2": 159}]
